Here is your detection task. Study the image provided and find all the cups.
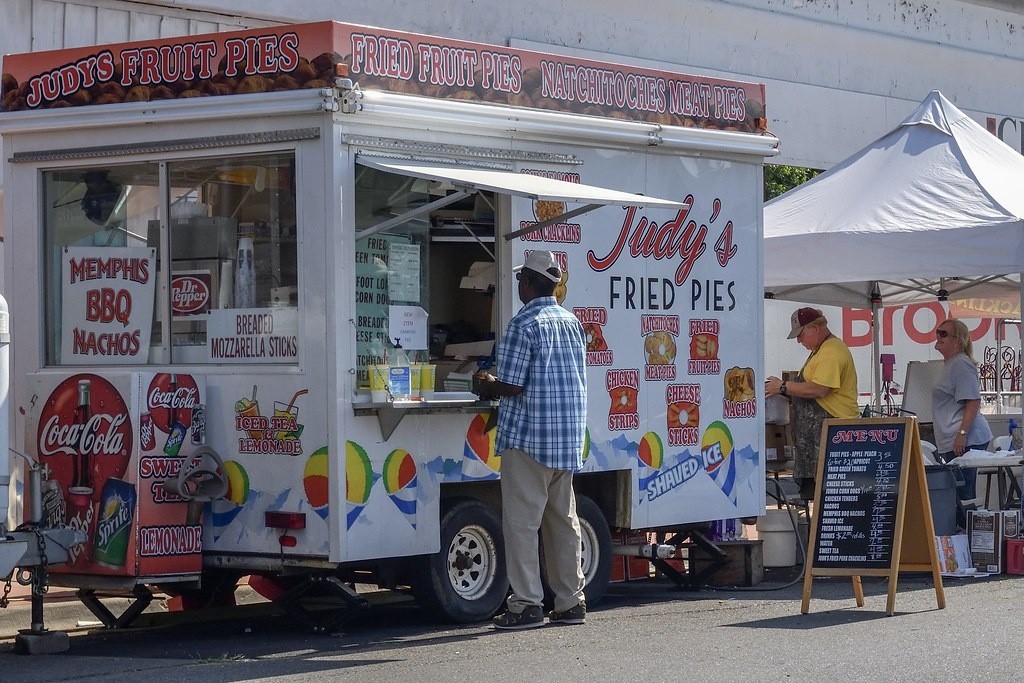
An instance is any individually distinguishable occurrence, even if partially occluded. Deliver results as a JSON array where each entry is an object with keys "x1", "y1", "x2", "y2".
[
  {"x1": 368, "y1": 365, "x2": 389, "y2": 402},
  {"x1": 234, "y1": 238, "x2": 256, "y2": 308},
  {"x1": 421, "y1": 364, "x2": 437, "y2": 401},
  {"x1": 411, "y1": 364, "x2": 421, "y2": 397}
]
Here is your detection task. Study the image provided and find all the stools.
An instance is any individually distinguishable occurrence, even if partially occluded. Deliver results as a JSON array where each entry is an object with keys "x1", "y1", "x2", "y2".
[{"x1": 978, "y1": 469, "x2": 1008, "y2": 510}]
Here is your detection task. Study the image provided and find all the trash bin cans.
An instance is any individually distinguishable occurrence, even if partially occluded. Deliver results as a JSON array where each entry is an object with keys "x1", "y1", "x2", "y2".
[{"x1": 898, "y1": 464, "x2": 966, "y2": 582}]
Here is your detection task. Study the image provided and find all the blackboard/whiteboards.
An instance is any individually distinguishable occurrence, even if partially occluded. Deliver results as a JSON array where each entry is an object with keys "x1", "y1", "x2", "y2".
[{"x1": 807, "y1": 416, "x2": 938, "y2": 576}]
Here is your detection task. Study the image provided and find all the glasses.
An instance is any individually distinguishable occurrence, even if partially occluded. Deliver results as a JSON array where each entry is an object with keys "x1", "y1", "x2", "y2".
[
  {"x1": 936, "y1": 328, "x2": 957, "y2": 338},
  {"x1": 515, "y1": 271, "x2": 534, "y2": 284}
]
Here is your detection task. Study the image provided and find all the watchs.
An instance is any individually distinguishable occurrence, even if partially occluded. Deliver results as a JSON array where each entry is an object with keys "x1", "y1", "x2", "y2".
[
  {"x1": 958, "y1": 429, "x2": 968, "y2": 435},
  {"x1": 780, "y1": 381, "x2": 787, "y2": 395}
]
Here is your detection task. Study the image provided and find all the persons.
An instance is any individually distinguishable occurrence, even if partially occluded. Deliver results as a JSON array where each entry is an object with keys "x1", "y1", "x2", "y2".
[
  {"x1": 932, "y1": 320, "x2": 994, "y2": 530},
  {"x1": 766, "y1": 307, "x2": 889, "y2": 584},
  {"x1": 471, "y1": 250, "x2": 587, "y2": 628}
]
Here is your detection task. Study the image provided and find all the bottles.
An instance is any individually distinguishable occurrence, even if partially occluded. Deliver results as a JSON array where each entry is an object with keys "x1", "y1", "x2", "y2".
[
  {"x1": 167, "y1": 374, "x2": 179, "y2": 427},
  {"x1": 73, "y1": 380, "x2": 94, "y2": 488},
  {"x1": 387, "y1": 337, "x2": 412, "y2": 401}
]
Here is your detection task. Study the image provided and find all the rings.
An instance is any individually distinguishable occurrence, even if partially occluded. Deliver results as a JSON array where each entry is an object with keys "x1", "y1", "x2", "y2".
[{"x1": 765, "y1": 391, "x2": 767, "y2": 394}]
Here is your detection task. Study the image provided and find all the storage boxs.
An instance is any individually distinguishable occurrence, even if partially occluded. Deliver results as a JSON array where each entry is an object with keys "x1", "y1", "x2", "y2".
[
  {"x1": 1006, "y1": 539, "x2": 1024, "y2": 575},
  {"x1": 765, "y1": 385, "x2": 804, "y2": 464},
  {"x1": 142, "y1": 214, "x2": 241, "y2": 346},
  {"x1": 687, "y1": 540, "x2": 766, "y2": 587}
]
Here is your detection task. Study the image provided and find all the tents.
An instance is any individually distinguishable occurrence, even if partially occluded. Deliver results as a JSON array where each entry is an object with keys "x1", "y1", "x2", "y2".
[{"x1": 763, "y1": 88, "x2": 1024, "y2": 418}]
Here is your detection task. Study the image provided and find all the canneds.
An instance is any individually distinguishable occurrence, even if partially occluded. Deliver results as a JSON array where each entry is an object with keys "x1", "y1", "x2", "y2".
[
  {"x1": 41, "y1": 477, "x2": 137, "y2": 570},
  {"x1": 162, "y1": 420, "x2": 187, "y2": 457},
  {"x1": 140, "y1": 411, "x2": 156, "y2": 451},
  {"x1": 191, "y1": 404, "x2": 206, "y2": 445}
]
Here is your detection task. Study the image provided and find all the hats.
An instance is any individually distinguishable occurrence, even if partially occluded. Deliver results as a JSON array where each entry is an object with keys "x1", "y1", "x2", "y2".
[
  {"x1": 512, "y1": 248, "x2": 563, "y2": 283},
  {"x1": 786, "y1": 307, "x2": 823, "y2": 339}
]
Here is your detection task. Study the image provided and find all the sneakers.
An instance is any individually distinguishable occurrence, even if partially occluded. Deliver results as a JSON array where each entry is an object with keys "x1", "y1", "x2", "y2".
[
  {"x1": 492, "y1": 604, "x2": 545, "y2": 630},
  {"x1": 548, "y1": 602, "x2": 587, "y2": 624}
]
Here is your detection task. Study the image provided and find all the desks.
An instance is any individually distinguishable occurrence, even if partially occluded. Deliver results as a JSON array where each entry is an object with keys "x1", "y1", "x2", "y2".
[{"x1": 954, "y1": 454, "x2": 1024, "y2": 508}]
